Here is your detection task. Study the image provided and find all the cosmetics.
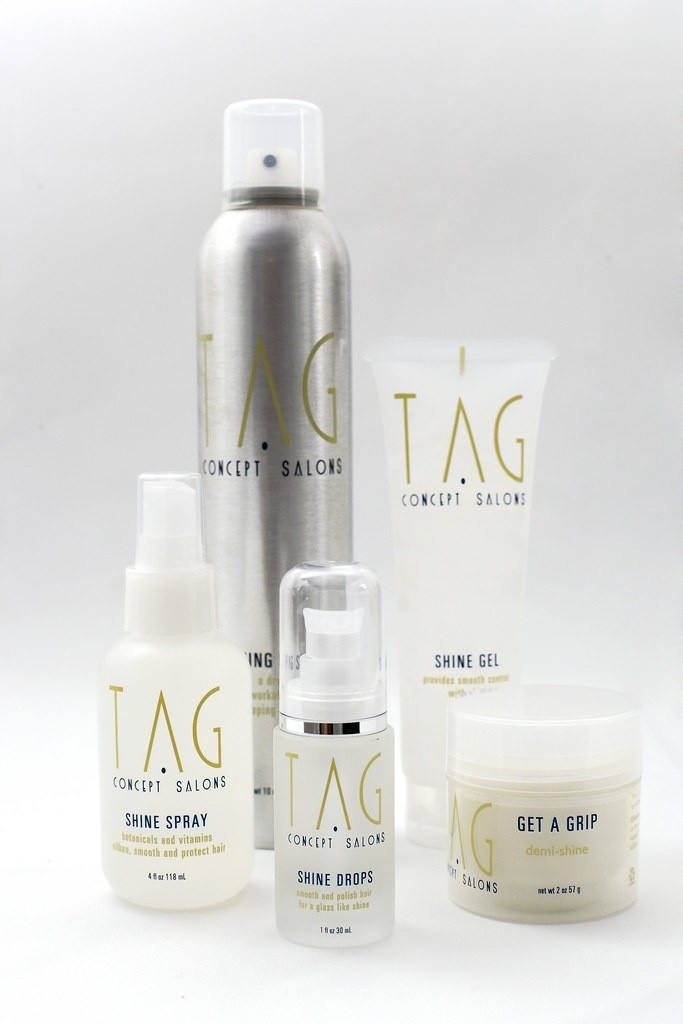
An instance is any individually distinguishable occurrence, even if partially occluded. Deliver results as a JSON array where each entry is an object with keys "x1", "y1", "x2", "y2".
[
  {"x1": 266, "y1": 551, "x2": 398, "y2": 950},
  {"x1": 443, "y1": 679, "x2": 641, "y2": 926},
  {"x1": 367, "y1": 333, "x2": 559, "y2": 748},
  {"x1": 98, "y1": 466, "x2": 255, "y2": 912},
  {"x1": 195, "y1": 92, "x2": 361, "y2": 854}
]
